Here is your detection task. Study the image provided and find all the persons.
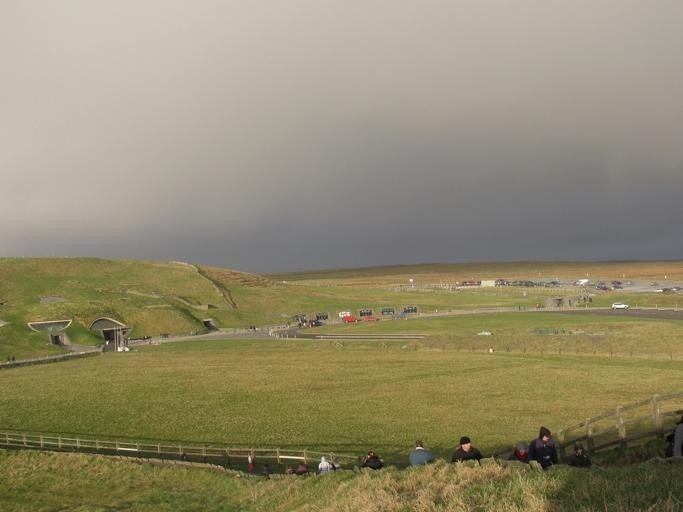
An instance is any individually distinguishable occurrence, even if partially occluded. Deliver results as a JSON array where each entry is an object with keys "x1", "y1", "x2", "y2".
[
  {"x1": 363, "y1": 450, "x2": 384, "y2": 470},
  {"x1": 567, "y1": 445, "x2": 592, "y2": 469},
  {"x1": 527, "y1": 426, "x2": 559, "y2": 470},
  {"x1": 508, "y1": 444, "x2": 531, "y2": 463},
  {"x1": 247, "y1": 457, "x2": 255, "y2": 473},
  {"x1": 263, "y1": 454, "x2": 342, "y2": 475},
  {"x1": 409, "y1": 439, "x2": 432, "y2": 465},
  {"x1": 453, "y1": 436, "x2": 484, "y2": 463}
]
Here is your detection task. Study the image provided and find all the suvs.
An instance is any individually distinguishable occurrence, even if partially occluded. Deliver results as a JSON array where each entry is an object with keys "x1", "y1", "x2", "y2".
[{"x1": 611, "y1": 302, "x2": 630, "y2": 310}]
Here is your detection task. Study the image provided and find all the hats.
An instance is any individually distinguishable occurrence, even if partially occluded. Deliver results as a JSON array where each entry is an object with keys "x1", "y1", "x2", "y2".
[{"x1": 460, "y1": 437, "x2": 471, "y2": 445}]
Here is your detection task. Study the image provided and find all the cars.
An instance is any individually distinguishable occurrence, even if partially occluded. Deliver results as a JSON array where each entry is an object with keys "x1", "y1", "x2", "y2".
[
  {"x1": 293, "y1": 306, "x2": 417, "y2": 328},
  {"x1": 454, "y1": 279, "x2": 681, "y2": 294}
]
